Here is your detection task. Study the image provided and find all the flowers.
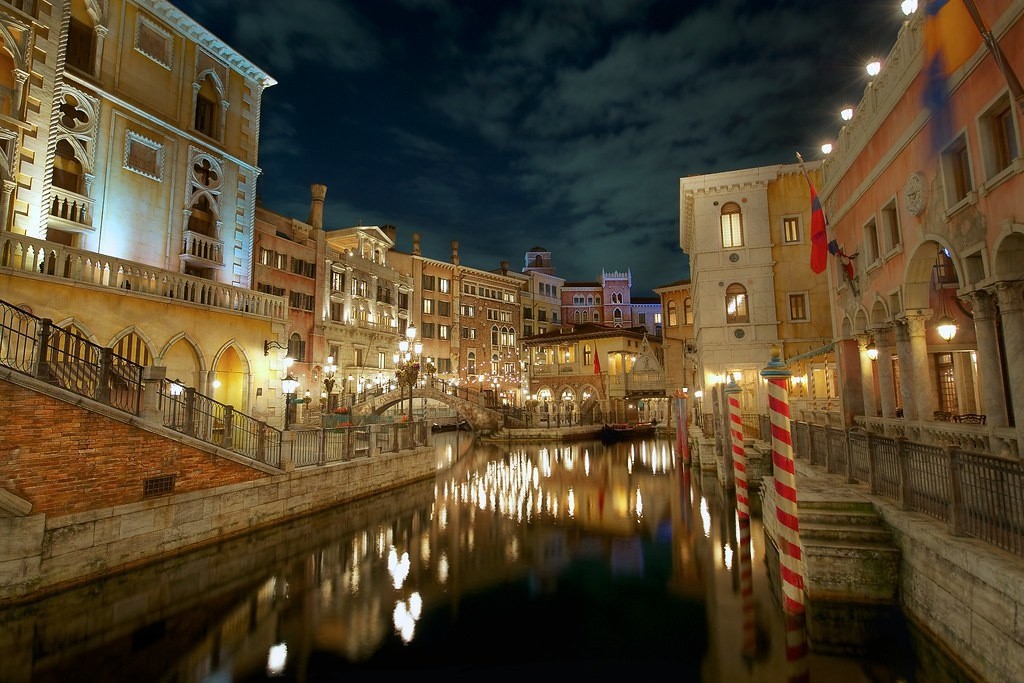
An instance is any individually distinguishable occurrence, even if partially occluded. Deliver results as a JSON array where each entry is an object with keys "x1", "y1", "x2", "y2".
[
  {"x1": 337, "y1": 423, "x2": 352, "y2": 432},
  {"x1": 334, "y1": 407, "x2": 348, "y2": 415},
  {"x1": 395, "y1": 362, "x2": 421, "y2": 389},
  {"x1": 402, "y1": 415, "x2": 409, "y2": 423}
]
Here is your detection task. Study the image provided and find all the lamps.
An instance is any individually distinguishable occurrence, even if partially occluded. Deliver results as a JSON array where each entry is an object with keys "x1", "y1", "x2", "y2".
[
  {"x1": 933, "y1": 241, "x2": 959, "y2": 343},
  {"x1": 865, "y1": 336, "x2": 879, "y2": 364},
  {"x1": 263, "y1": 340, "x2": 294, "y2": 365}
]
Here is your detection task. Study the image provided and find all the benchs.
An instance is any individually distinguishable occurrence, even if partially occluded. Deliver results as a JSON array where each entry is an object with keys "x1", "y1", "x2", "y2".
[{"x1": 934, "y1": 410, "x2": 986, "y2": 424}]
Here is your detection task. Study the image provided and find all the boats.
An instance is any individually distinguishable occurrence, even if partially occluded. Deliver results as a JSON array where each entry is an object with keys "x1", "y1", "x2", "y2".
[{"x1": 602, "y1": 419, "x2": 662, "y2": 437}]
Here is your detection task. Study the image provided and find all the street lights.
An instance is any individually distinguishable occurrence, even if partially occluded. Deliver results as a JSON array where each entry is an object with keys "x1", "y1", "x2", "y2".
[
  {"x1": 393, "y1": 321, "x2": 423, "y2": 421},
  {"x1": 281, "y1": 375, "x2": 299, "y2": 431},
  {"x1": 427, "y1": 357, "x2": 435, "y2": 379},
  {"x1": 359, "y1": 371, "x2": 398, "y2": 401},
  {"x1": 695, "y1": 391, "x2": 703, "y2": 431},
  {"x1": 449, "y1": 378, "x2": 460, "y2": 397},
  {"x1": 499, "y1": 391, "x2": 512, "y2": 414},
  {"x1": 305, "y1": 390, "x2": 311, "y2": 410},
  {"x1": 526, "y1": 394, "x2": 538, "y2": 427},
  {"x1": 170, "y1": 380, "x2": 183, "y2": 429},
  {"x1": 324, "y1": 356, "x2": 339, "y2": 413},
  {"x1": 491, "y1": 378, "x2": 500, "y2": 406},
  {"x1": 541, "y1": 390, "x2": 551, "y2": 428},
  {"x1": 561, "y1": 390, "x2": 573, "y2": 428},
  {"x1": 347, "y1": 374, "x2": 355, "y2": 395},
  {"x1": 320, "y1": 391, "x2": 326, "y2": 422},
  {"x1": 583, "y1": 391, "x2": 593, "y2": 425}
]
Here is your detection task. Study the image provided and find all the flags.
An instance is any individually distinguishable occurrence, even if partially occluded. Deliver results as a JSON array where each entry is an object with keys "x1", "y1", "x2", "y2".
[
  {"x1": 810, "y1": 183, "x2": 828, "y2": 274},
  {"x1": 592, "y1": 343, "x2": 603, "y2": 375}
]
